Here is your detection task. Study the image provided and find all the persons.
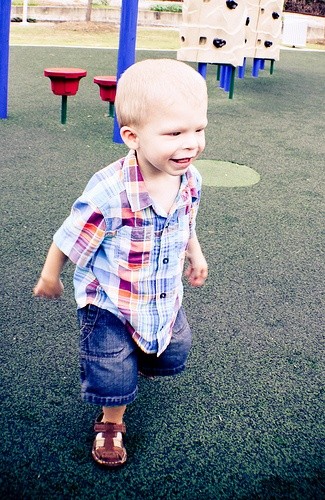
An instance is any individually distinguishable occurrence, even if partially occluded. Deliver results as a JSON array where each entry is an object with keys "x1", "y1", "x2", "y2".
[{"x1": 33, "y1": 58, "x2": 209, "y2": 468}]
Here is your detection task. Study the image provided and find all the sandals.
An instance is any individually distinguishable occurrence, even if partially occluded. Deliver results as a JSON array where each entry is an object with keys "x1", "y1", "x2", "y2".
[{"x1": 92, "y1": 411, "x2": 127, "y2": 466}]
